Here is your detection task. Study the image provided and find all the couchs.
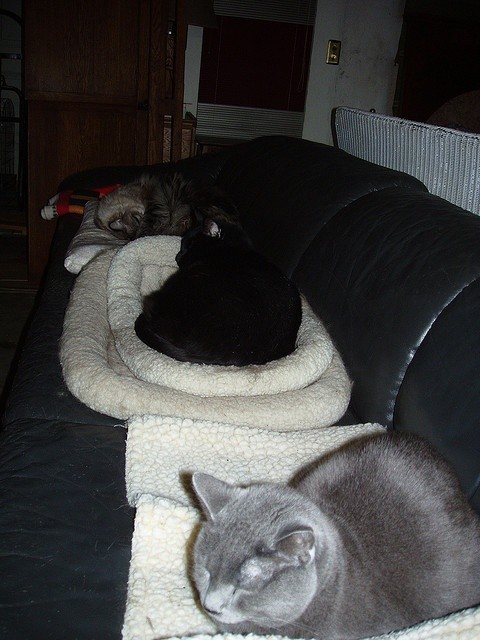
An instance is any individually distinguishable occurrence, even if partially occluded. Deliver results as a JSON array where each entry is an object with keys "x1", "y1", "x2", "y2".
[{"x1": 1, "y1": 127, "x2": 480, "y2": 634}]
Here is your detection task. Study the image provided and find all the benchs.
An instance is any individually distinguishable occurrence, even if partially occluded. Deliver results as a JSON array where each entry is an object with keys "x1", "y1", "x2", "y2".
[{"x1": 195, "y1": 103, "x2": 307, "y2": 157}]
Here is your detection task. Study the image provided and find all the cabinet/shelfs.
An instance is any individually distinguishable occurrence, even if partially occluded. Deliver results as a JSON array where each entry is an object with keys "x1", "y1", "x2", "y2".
[{"x1": 21, "y1": 0, "x2": 164, "y2": 287}]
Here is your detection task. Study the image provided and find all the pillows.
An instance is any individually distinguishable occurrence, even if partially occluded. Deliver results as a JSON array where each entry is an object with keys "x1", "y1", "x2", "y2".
[{"x1": 65, "y1": 198, "x2": 132, "y2": 274}]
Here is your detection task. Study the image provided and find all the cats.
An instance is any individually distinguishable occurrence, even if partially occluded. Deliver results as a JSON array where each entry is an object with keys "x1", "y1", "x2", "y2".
[
  {"x1": 141, "y1": 187, "x2": 303, "y2": 366},
  {"x1": 191, "y1": 428, "x2": 480, "y2": 640}
]
